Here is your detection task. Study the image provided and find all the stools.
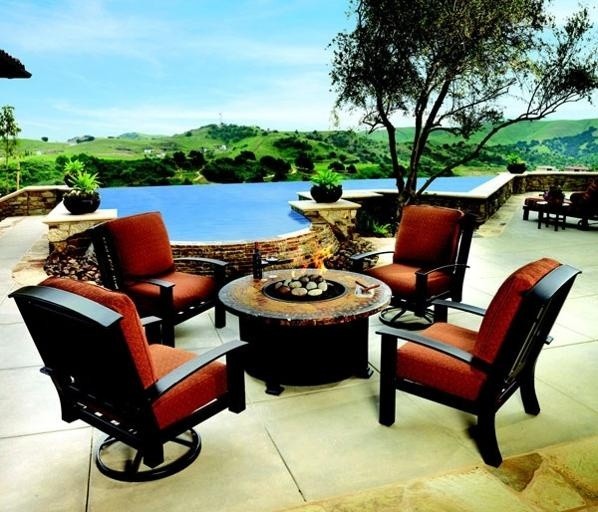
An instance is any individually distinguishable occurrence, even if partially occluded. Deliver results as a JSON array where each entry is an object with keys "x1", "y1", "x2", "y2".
[{"x1": 534, "y1": 199, "x2": 572, "y2": 232}]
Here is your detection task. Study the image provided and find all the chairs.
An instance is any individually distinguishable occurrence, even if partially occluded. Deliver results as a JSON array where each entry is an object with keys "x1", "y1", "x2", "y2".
[
  {"x1": 520, "y1": 174, "x2": 598, "y2": 232},
  {"x1": 369, "y1": 253, "x2": 586, "y2": 473},
  {"x1": 342, "y1": 200, "x2": 481, "y2": 332},
  {"x1": 1, "y1": 272, "x2": 256, "y2": 486},
  {"x1": 86, "y1": 205, "x2": 234, "y2": 357}
]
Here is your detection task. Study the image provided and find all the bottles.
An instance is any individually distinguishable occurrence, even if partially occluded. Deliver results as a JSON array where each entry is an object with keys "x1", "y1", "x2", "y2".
[{"x1": 252, "y1": 242, "x2": 263, "y2": 280}]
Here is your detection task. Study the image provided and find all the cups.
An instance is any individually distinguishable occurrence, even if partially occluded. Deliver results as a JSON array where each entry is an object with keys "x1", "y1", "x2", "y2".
[
  {"x1": 261, "y1": 270, "x2": 268, "y2": 281},
  {"x1": 269, "y1": 265, "x2": 278, "y2": 278}
]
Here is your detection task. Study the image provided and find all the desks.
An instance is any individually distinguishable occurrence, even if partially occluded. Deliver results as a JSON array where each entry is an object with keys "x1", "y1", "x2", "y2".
[{"x1": 214, "y1": 265, "x2": 394, "y2": 398}]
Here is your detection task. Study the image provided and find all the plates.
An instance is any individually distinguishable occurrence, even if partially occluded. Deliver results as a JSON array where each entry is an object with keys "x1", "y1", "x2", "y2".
[
  {"x1": 260, "y1": 261, "x2": 268, "y2": 265},
  {"x1": 265, "y1": 258, "x2": 280, "y2": 262}
]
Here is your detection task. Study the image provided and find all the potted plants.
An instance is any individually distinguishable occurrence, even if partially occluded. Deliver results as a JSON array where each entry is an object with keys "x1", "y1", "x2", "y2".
[
  {"x1": 535, "y1": 182, "x2": 566, "y2": 208},
  {"x1": 59, "y1": 167, "x2": 104, "y2": 217},
  {"x1": 307, "y1": 160, "x2": 347, "y2": 207},
  {"x1": 61, "y1": 158, "x2": 90, "y2": 189}
]
